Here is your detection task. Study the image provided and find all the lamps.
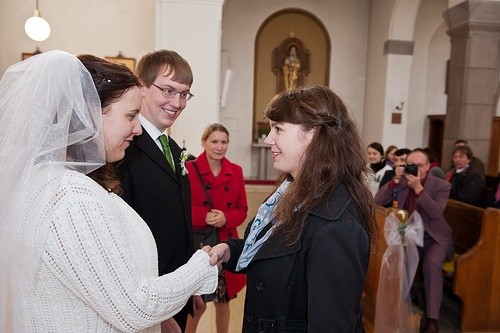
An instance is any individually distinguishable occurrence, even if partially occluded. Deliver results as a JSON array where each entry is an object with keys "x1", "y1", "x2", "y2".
[{"x1": 23, "y1": 0, "x2": 51, "y2": 42}]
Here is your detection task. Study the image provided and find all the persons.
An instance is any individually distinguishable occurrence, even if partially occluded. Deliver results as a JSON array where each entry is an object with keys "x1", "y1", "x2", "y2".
[
  {"x1": 0, "y1": 51, "x2": 222, "y2": 333},
  {"x1": 282, "y1": 47, "x2": 301, "y2": 91},
  {"x1": 178, "y1": 122, "x2": 249, "y2": 333},
  {"x1": 359, "y1": 138, "x2": 500, "y2": 333},
  {"x1": 110, "y1": 49, "x2": 193, "y2": 333},
  {"x1": 208, "y1": 84, "x2": 378, "y2": 333}
]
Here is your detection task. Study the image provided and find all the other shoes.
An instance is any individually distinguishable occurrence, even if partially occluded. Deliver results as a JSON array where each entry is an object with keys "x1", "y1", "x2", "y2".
[{"x1": 424, "y1": 322, "x2": 439, "y2": 333}]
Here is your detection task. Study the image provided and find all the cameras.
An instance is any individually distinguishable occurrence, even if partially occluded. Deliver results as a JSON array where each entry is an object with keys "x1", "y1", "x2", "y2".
[{"x1": 404, "y1": 163, "x2": 417, "y2": 176}]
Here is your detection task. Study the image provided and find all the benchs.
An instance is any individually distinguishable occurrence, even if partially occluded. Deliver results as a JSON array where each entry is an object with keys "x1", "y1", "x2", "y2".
[{"x1": 443, "y1": 174, "x2": 500, "y2": 333}]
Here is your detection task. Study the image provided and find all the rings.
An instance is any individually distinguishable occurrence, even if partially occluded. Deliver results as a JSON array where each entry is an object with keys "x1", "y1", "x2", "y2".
[{"x1": 214, "y1": 252, "x2": 220, "y2": 260}]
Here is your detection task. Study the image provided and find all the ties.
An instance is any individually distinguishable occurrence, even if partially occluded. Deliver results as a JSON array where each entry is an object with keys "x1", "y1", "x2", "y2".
[{"x1": 158, "y1": 134, "x2": 175, "y2": 178}]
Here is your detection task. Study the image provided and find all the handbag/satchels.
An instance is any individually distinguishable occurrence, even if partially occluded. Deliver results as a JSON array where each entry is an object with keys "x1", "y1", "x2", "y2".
[{"x1": 192, "y1": 227, "x2": 219, "y2": 254}]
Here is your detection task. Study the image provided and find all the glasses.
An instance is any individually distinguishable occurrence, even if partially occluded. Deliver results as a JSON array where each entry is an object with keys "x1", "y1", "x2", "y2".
[
  {"x1": 152, "y1": 83, "x2": 195, "y2": 101},
  {"x1": 418, "y1": 164, "x2": 426, "y2": 168}
]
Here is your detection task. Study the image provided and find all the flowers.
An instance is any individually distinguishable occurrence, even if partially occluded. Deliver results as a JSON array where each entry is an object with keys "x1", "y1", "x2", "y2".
[{"x1": 180, "y1": 150, "x2": 192, "y2": 176}]
